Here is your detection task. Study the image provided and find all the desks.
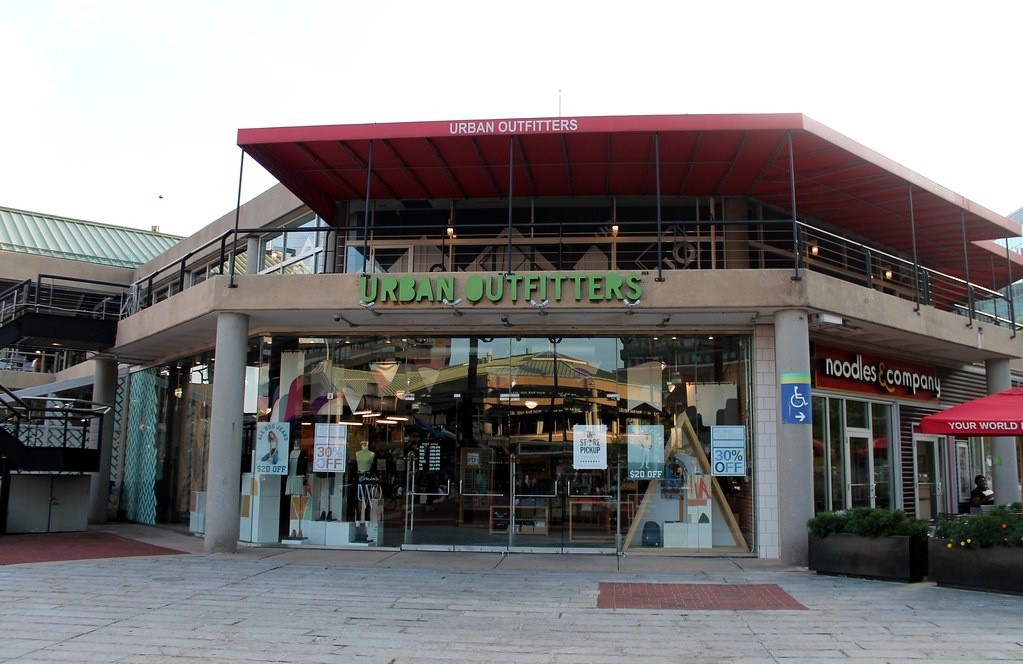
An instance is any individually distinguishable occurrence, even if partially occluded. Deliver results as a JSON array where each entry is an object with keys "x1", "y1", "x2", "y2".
[{"x1": 568, "y1": 500, "x2": 634, "y2": 545}]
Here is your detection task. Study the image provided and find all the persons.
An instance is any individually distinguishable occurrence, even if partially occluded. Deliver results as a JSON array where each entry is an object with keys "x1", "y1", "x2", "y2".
[
  {"x1": 31, "y1": 358, "x2": 41, "y2": 371},
  {"x1": 384, "y1": 449, "x2": 402, "y2": 482},
  {"x1": 918, "y1": 456, "x2": 932, "y2": 482},
  {"x1": 969, "y1": 475, "x2": 994, "y2": 514},
  {"x1": 350, "y1": 442, "x2": 381, "y2": 485},
  {"x1": 638, "y1": 433, "x2": 652, "y2": 469},
  {"x1": 261, "y1": 431, "x2": 279, "y2": 464},
  {"x1": 285, "y1": 440, "x2": 308, "y2": 495}
]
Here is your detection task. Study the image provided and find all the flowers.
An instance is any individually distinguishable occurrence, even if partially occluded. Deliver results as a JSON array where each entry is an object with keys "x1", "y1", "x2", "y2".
[{"x1": 933, "y1": 501, "x2": 1023, "y2": 551}]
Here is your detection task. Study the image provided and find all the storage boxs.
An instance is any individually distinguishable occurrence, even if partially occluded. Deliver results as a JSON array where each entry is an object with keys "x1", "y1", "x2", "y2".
[{"x1": 521, "y1": 525, "x2": 546, "y2": 533}]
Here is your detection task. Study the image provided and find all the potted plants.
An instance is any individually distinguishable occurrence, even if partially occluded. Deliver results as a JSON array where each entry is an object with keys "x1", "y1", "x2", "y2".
[{"x1": 808, "y1": 508, "x2": 929, "y2": 585}]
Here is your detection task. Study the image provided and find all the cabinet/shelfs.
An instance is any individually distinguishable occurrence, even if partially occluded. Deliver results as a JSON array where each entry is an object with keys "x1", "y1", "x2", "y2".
[{"x1": 490, "y1": 505, "x2": 551, "y2": 537}]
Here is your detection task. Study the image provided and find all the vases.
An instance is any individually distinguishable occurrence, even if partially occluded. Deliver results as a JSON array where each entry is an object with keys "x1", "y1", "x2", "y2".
[{"x1": 931, "y1": 540, "x2": 1023, "y2": 595}]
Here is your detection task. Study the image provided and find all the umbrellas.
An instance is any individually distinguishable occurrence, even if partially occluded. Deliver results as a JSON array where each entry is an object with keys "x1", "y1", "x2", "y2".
[
  {"x1": 920, "y1": 388, "x2": 1023, "y2": 437},
  {"x1": 813, "y1": 439, "x2": 834, "y2": 457},
  {"x1": 852, "y1": 437, "x2": 888, "y2": 458}
]
